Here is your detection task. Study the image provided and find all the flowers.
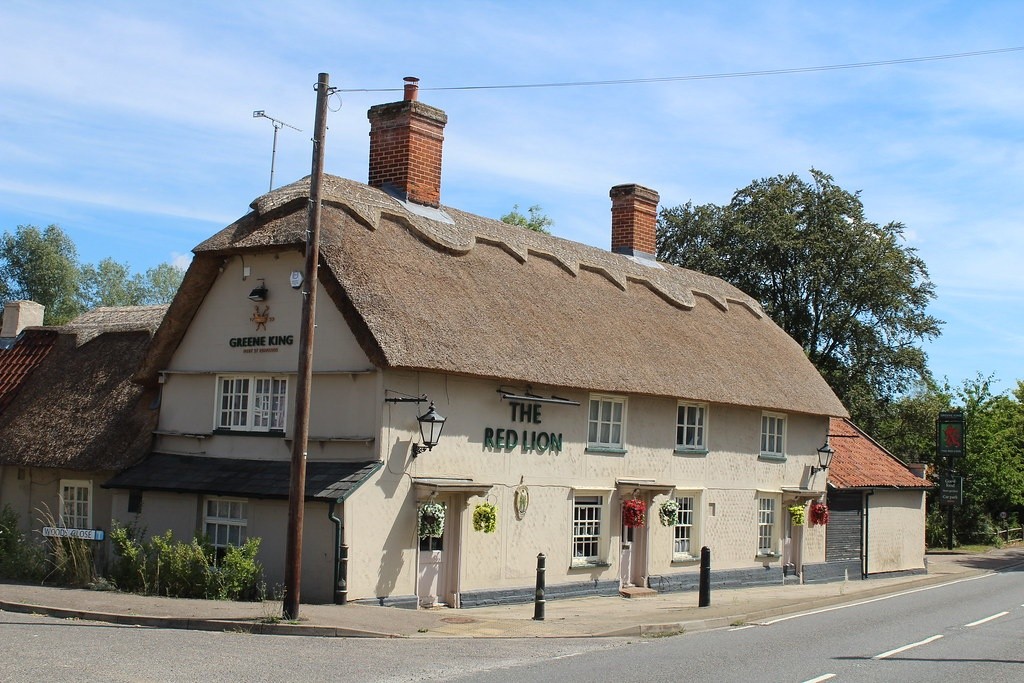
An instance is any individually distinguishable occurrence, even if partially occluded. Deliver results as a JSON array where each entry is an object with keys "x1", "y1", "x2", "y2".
[
  {"x1": 473, "y1": 502, "x2": 498, "y2": 533},
  {"x1": 417, "y1": 503, "x2": 448, "y2": 538},
  {"x1": 658, "y1": 500, "x2": 680, "y2": 526},
  {"x1": 624, "y1": 500, "x2": 646, "y2": 528},
  {"x1": 784, "y1": 502, "x2": 806, "y2": 527},
  {"x1": 810, "y1": 504, "x2": 829, "y2": 526}
]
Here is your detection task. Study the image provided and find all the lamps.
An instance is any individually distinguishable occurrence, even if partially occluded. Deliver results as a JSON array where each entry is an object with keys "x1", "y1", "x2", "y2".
[
  {"x1": 811, "y1": 441, "x2": 836, "y2": 475},
  {"x1": 412, "y1": 401, "x2": 448, "y2": 458},
  {"x1": 248, "y1": 282, "x2": 268, "y2": 302}
]
